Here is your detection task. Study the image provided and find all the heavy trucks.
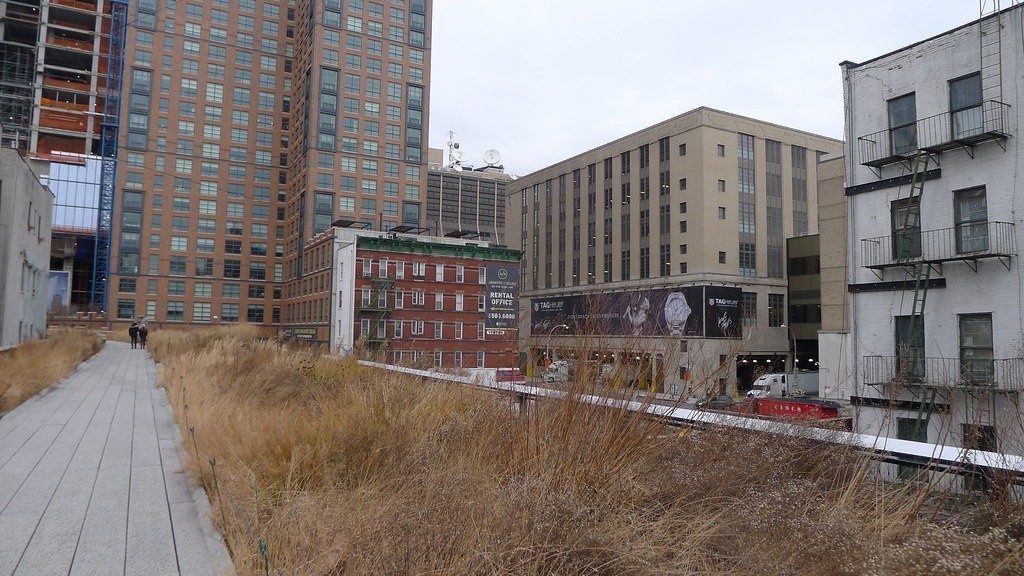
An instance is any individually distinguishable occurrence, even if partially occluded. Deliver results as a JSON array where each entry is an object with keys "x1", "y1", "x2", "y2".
[
  {"x1": 540, "y1": 360, "x2": 599, "y2": 383},
  {"x1": 745, "y1": 371, "x2": 819, "y2": 400}
]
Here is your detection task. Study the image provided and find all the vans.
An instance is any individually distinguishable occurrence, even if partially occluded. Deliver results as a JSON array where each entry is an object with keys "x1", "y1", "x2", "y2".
[{"x1": 496, "y1": 370, "x2": 528, "y2": 385}]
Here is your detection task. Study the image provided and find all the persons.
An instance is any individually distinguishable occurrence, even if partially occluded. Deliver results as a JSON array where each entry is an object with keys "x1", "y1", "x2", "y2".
[{"x1": 129, "y1": 321, "x2": 149, "y2": 350}]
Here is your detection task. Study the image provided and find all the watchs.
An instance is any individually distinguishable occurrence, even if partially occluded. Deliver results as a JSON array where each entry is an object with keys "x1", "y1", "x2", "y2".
[
  {"x1": 716, "y1": 306, "x2": 733, "y2": 337},
  {"x1": 624, "y1": 294, "x2": 653, "y2": 336},
  {"x1": 665, "y1": 293, "x2": 691, "y2": 335}
]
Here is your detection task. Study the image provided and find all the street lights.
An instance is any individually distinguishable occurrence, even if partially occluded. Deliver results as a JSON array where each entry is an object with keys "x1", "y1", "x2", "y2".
[
  {"x1": 547, "y1": 324, "x2": 570, "y2": 358},
  {"x1": 781, "y1": 325, "x2": 799, "y2": 388}
]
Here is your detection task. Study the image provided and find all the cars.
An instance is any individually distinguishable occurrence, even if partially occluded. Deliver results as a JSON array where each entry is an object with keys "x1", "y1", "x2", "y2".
[{"x1": 695, "y1": 395, "x2": 735, "y2": 410}]
